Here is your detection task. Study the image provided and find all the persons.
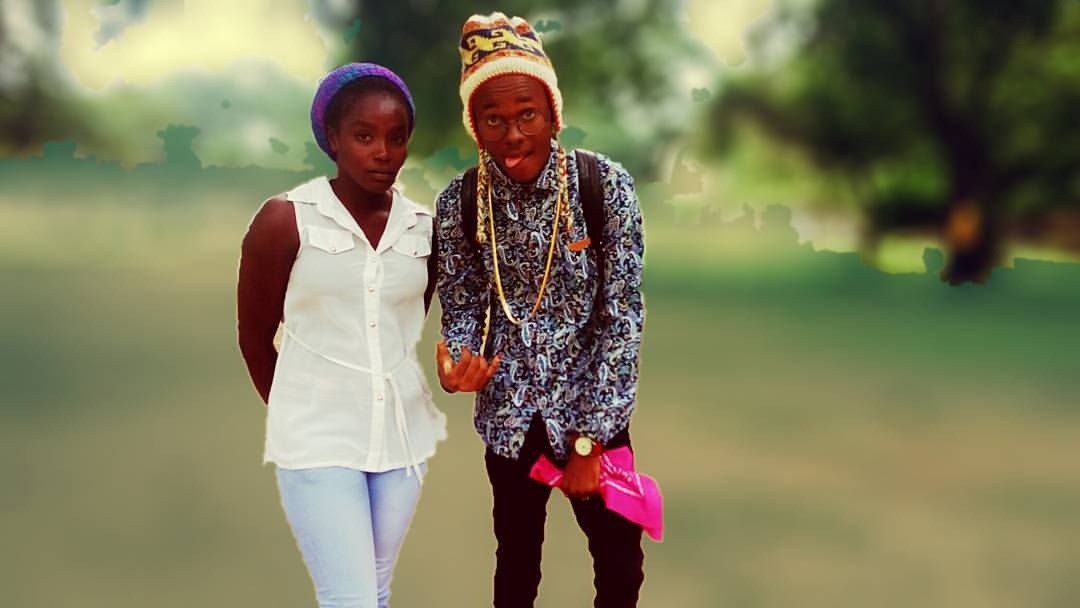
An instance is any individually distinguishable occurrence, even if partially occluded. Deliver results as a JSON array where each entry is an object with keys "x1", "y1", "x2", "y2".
[
  {"x1": 431, "y1": 13, "x2": 645, "y2": 608},
  {"x1": 237, "y1": 63, "x2": 448, "y2": 608}
]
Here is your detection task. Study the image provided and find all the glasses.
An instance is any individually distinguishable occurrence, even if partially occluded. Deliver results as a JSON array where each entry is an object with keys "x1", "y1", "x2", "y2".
[{"x1": 477, "y1": 111, "x2": 549, "y2": 142}]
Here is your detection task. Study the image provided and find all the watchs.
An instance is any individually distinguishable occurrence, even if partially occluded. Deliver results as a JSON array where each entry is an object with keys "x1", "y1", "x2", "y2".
[{"x1": 566, "y1": 434, "x2": 603, "y2": 459}]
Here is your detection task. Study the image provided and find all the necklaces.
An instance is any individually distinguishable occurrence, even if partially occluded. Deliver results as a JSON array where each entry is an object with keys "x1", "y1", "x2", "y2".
[{"x1": 485, "y1": 152, "x2": 564, "y2": 328}]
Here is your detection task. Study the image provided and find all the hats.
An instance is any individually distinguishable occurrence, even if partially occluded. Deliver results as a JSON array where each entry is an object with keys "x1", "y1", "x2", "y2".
[
  {"x1": 310, "y1": 63, "x2": 416, "y2": 161},
  {"x1": 458, "y1": 11, "x2": 574, "y2": 244}
]
